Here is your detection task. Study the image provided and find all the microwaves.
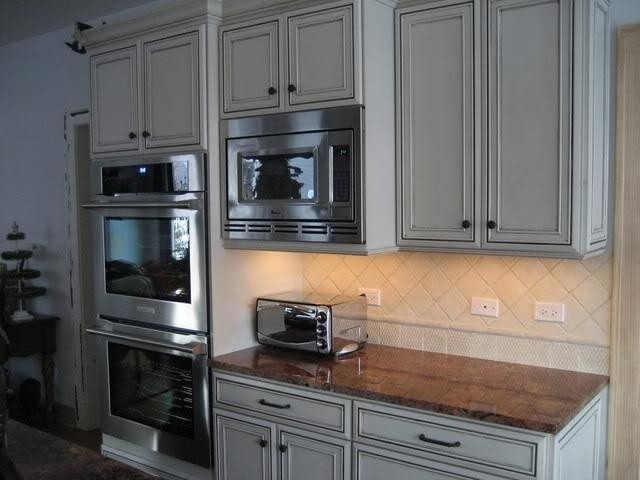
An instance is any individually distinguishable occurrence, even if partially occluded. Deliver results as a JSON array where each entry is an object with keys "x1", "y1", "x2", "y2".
[
  {"x1": 218, "y1": 105, "x2": 367, "y2": 243},
  {"x1": 254, "y1": 349, "x2": 371, "y2": 393},
  {"x1": 254, "y1": 291, "x2": 373, "y2": 357}
]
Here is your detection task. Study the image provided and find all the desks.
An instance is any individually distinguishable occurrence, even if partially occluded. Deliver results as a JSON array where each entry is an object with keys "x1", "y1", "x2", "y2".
[{"x1": 0, "y1": 313, "x2": 63, "y2": 419}]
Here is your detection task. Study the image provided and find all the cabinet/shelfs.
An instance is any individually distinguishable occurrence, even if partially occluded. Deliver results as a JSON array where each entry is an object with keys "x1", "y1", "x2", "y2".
[
  {"x1": 532, "y1": 301, "x2": 565, "y2": 323},
  {"x1": 216, "y1": 1, "x2": 397, "y2": 119},
  {"x1": 209, "y1": 371, "x2": 382, "y2": 480},
  {"x1": 88, "y1": 15, "x2": 206, "y2": 160},
  {"x1": 396, "y1": 1, "x2": 618, "y2": 257},
  {"x1": 353, "y1": 384, "x2": 610, "y2": 480}
]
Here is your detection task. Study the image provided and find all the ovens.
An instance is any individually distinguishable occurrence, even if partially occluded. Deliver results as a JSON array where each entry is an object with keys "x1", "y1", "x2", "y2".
[{"x1": 79, "y1": 150, "x2": 213, "y2": 467}]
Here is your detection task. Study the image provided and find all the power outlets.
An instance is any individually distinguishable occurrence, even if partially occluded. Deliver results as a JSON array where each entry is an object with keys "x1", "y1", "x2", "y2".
[{"x1": 359, "y1": 288, "x2": 380, "y2": 306}]
